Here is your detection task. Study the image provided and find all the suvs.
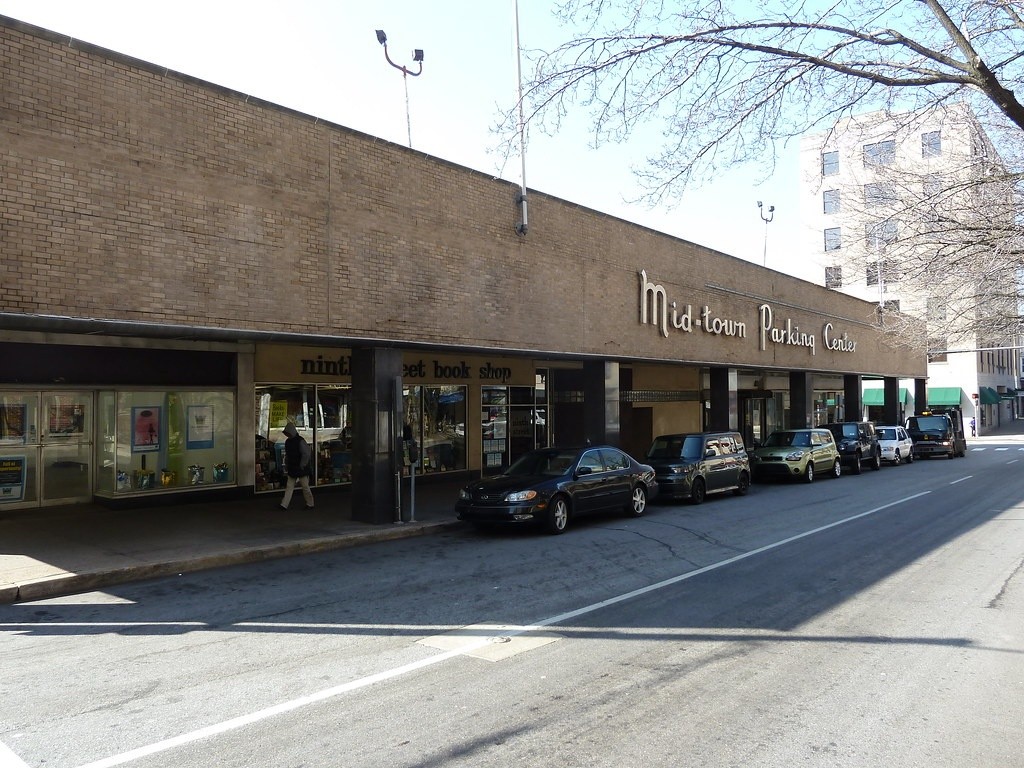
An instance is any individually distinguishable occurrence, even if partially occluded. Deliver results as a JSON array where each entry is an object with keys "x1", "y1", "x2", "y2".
[
  {"x1": 644, "y1": 431, "x2": 753, "y2": 506},
  {"x1": 903, "y1": 410, "x2": 957, "y2": 460},
  {"x1": 816, "y1": 421, "x2": 882, "y2": 475}
]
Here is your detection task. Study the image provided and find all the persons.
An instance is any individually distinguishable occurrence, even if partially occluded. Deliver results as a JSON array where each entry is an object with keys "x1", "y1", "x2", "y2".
[
  {"x1": 970, "y1": 417, "x2": 975, "y2": 437},
  {"x1": 277, "y1": 422, "x2": 314, "y2": 510},
  {"x1": 337, "y1": 419, "x2": 351, "y2": 449}
]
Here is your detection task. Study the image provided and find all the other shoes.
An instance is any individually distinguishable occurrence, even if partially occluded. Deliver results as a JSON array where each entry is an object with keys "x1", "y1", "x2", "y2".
[
  {"x1": 303, "y1": 506, "x2": 314, "y2": 511},
  {"x1": 274, "y1": 504, "x2": 286, "y2": 511}
]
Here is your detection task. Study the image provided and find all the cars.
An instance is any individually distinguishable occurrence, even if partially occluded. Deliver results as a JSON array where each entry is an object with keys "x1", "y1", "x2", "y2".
[
  {"x1": 750, "y1": 428, "x2": 843, "y2": 483},
  {"x1": 876, "y1": 425, "x2": 915, "y2": 466},
  {"x1": 453, "y1": 440, "x2": 660, "y2": 536}
]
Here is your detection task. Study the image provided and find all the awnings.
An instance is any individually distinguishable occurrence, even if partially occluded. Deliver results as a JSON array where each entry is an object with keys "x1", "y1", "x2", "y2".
[
  {"x1": 980, "y1": 387, "x2": 1001, "y2": 404},
  {"x1": 862, "y1": 388, "x2": 908, "y2": 405},
  {"x1": 1002, "y1": 388, "x2": 1018, "y2": 400},
  {"x1": 927, "y1": 387, "x2": 961, "y2": 405}
]
